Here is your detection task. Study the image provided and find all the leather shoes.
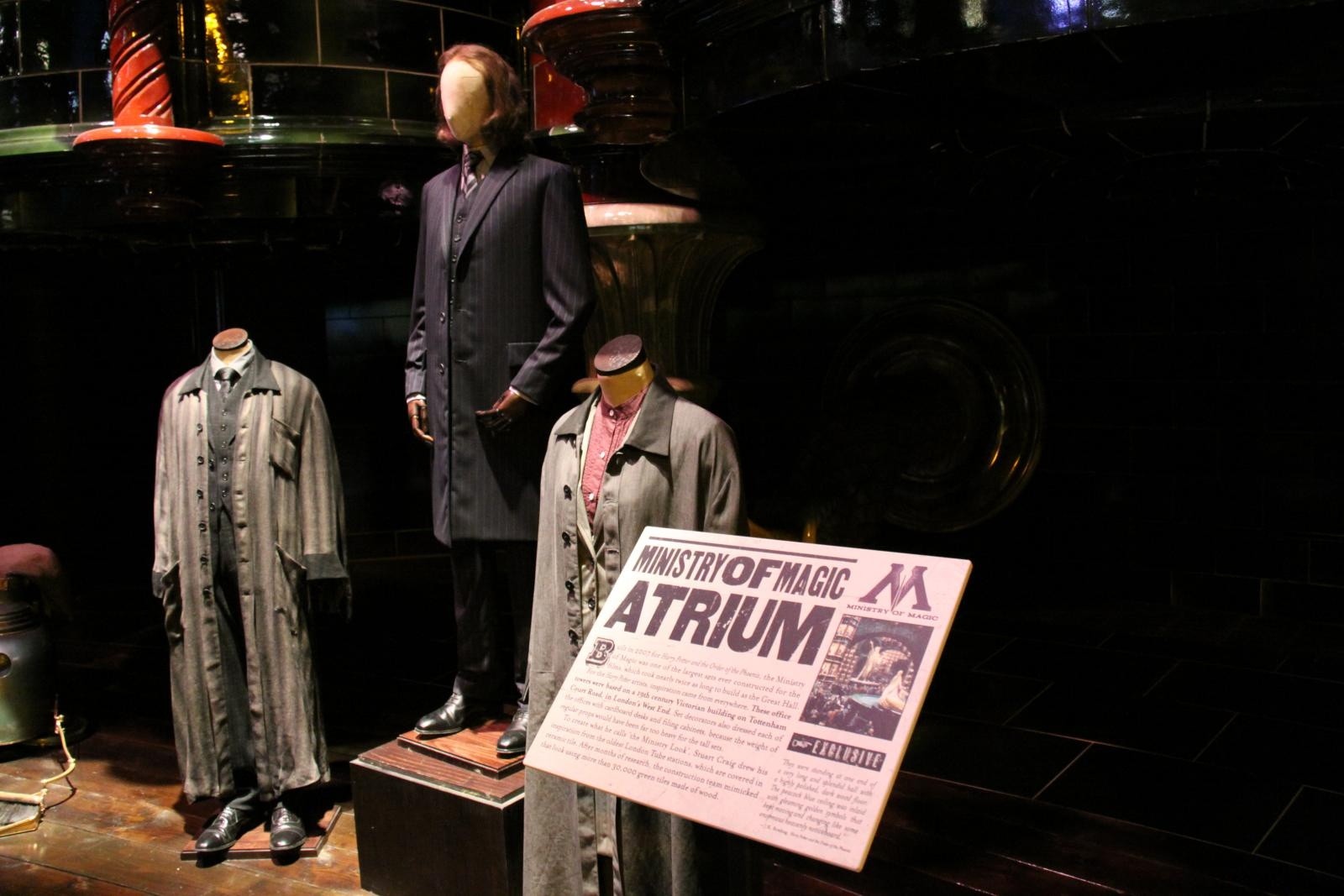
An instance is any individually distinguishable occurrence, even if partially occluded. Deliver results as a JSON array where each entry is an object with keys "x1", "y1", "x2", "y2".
[
  {"x1": 194, "y1": 806, "x2": 256, "y2": 855},
  {"x1": 497, "y1": 704, "x2": 529, "y2": 756},
  {"x1": 270, "y1": 807, "x2": 306, "y2": 854},
  {"x1": 416, "y1": 676, "x2": 504, "y2": 737}
]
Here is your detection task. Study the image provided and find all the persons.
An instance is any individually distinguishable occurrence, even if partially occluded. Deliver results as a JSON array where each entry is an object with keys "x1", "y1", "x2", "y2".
[
  {"x1": 401, "y1": 44, "x2": 592, "y2": 762},
  {"x1": 151, "y1": 326, "x2": 355, "y2": 852},
  {"x1": 798, "y1": 622, "x2": 930, "y2": 742},
  {"x1": 523, "y1": 332, "x2": 743, "y2": 894}
]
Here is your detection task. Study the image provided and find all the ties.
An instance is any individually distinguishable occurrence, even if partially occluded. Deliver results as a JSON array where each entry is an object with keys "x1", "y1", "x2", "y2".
[
  {"x1": 459, "y1": 151, "x2": 485, "y2": 198},
  {"x1": 215, "y1": 368, "x2": 240, "y2": 400}
]
